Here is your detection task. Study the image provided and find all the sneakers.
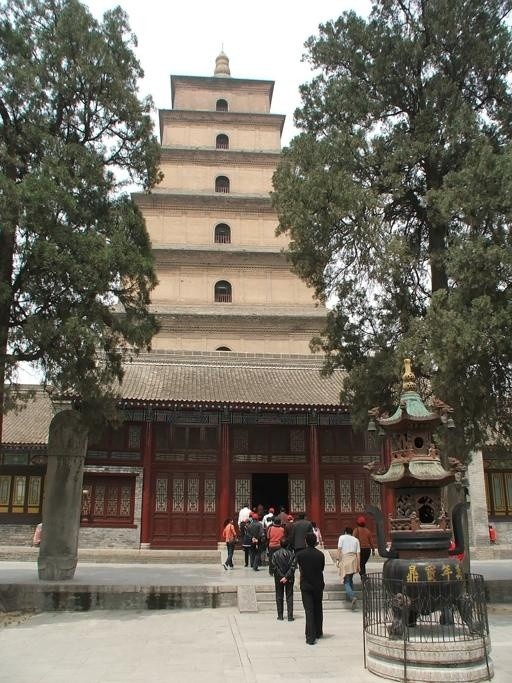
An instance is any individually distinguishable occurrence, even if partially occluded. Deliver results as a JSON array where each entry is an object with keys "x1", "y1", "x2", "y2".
[
  {"x1": 351, "y1": 597, "x2": 357, "y2": 610},
  {"x1": 224, "y1": 564, "x2": 228, "y2": 570},
  {"x1": 230, "y1": 566, "x2": 232, "y2": 570}
]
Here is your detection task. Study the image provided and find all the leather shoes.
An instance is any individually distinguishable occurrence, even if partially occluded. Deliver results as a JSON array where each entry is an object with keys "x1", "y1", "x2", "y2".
[
  {"x1": 288, "y1": 617, "x2": 294, "y2": 621},
  {"x1": 277, "y1": 617, "x2": 283, "y2": 620}
]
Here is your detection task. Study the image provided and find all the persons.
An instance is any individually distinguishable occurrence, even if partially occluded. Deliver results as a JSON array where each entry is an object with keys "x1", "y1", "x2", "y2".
[
  {"x1": 488, "y1": 523, "x2": 498, "y2": 545},
  {"x1": 297, "y1": 533, "x2": 325, "y2": 645},
  {"x1": 237, "y1": 502, "x2": 324, "y2": 576},
  {"x1": 272, "y1": 538, "x2": 298, "y2": 622},
  {"x1": 352, "y1": 517, "x2": 375, "y2": 592},
  {"x1": 336, "y1": 528, "x2": 361, "y2": 610},
  {"x1": 222, "y1": 517, "x2": 238, "y2": 570},
  {"x1": 448, "y1": 538, "x2": 464, "y2": 561}
]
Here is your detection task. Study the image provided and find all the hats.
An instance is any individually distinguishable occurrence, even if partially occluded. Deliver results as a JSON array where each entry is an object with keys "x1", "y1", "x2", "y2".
[
  {"x1": 249, "y1": 508, "x2": 294, "y2": 520},
  {"x1": 357, "y1": 515, "x2": 366, "y2": 526}
]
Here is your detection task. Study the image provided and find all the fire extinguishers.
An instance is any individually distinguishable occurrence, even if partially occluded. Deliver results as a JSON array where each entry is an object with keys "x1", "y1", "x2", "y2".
[{"x1": 489, "y1": 525, "x2": 495, "y2": 544}]
